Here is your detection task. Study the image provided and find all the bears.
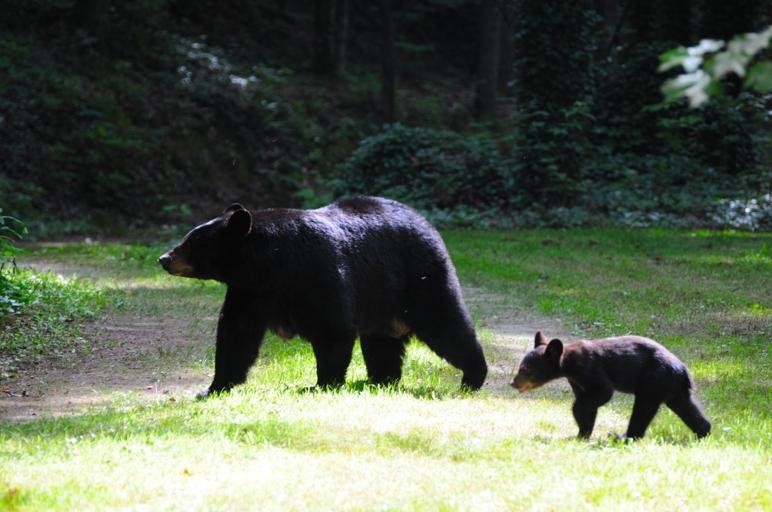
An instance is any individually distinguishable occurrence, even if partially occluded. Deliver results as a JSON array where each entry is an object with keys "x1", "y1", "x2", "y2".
[
  {"x1": 157, "y1": 194, "x2": 488, "y2": 402},
  {"x1": 508, "y1": 331, "x2": 711, "y2": 446}
]
